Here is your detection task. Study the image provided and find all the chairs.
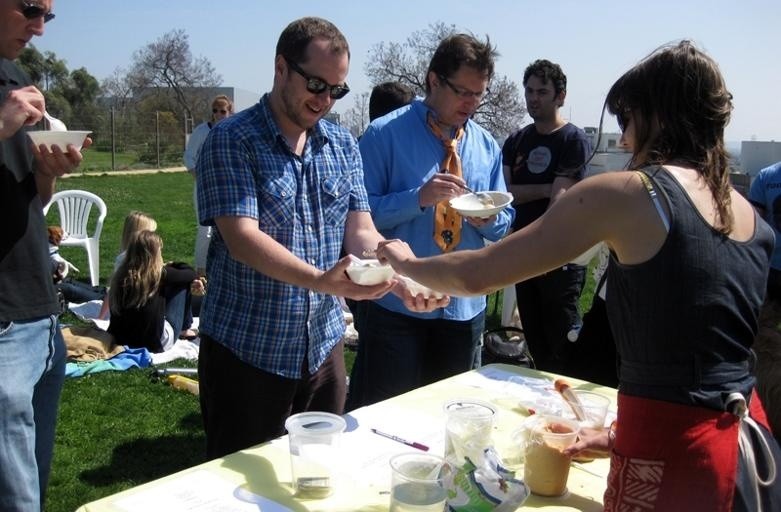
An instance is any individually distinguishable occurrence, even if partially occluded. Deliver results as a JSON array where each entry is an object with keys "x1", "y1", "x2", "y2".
[{"x1": 40, "y1": 188, "x2": 110, "y2": 289}]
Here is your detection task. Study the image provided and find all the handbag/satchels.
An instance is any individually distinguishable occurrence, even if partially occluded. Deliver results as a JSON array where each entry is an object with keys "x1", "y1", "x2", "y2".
[
  {"x1": 472, "y1": 321, "x2": 535, "y2": 368},
  {"x1": 60, "y1": 326, "x2": 114, "y2": 363},
  {"x1": 570, "y1": 265, "x2": 614, "y2": 389}
]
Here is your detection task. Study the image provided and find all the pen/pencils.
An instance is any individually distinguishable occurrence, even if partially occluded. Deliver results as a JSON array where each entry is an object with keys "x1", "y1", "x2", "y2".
[{"x1": 371, "y1": 429, "x2": 429, "y2": 451}]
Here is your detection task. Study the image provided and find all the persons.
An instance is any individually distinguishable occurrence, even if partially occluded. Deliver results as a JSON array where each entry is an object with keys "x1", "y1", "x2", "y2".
[
  {"x1": 107, "y1": 210, "x2": 204, "y2": 317},
  {"x1": 47, "y1": 225, "x2": 108, "y2": 304},
  {"x1": 343, "y1": 80, "x2": 416, "y2": 413},
  {"x1": 106, "y1": 231, "x2": 204, "y2": 352},
  {"x1": 500, "y1": 61, "x2": 594, "y2": 377},
  {"x1": 747, "y1": 160, "x2": 780, "y2": 446},
  {"x1": 372, "y1": 36, "x2": 778, "y2": 512},
  {"x1": 196, "y1": 18, "x2": 453, "y2": 456},
  {"x1": 0, "y1": 0, "x2": 97, "y2": 512},
  {"x1": 336, "y1": 33, "x2": 517, "y2": 413},
  {"x1": 184, "y1": 95, "x2": 233, "y2": 276}
]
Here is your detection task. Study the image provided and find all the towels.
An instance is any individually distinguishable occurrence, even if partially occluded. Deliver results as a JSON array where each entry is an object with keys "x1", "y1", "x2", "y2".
[{"x1": 67, "y1": 299, "x2": 202, "y2": 366}]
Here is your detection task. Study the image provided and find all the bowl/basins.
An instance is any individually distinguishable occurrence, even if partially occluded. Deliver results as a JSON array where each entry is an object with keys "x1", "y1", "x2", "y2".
[{"x1": 341, "y1": 256, "x2": 398, "y2": 287}]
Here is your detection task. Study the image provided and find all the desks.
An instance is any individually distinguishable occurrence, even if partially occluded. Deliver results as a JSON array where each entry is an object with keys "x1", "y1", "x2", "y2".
[{"x1": 76, "y1": 361, "x2": 620, "y2": 512}]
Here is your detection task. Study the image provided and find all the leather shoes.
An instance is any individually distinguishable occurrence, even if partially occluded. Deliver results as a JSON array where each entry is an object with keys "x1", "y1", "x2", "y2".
[{"x1": 181, "y1": 329, "x2": 200, "y2": 341}]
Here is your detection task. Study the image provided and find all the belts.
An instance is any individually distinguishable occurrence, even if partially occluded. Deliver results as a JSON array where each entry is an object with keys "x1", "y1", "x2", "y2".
[{"x1": 552, "y1": 264, "x2": 586, "y2": 272}]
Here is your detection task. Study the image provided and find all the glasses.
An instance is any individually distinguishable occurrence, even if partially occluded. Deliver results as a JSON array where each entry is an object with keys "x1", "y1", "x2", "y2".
[
  {"x1": 212, "y1": 108, "x2": 228, "y2": 116},
  {"x1": 283, "y1": 55, "x2": 350, "y2": 99},
  {"x1": 19, "y1": 0, "x2": 55, "y2": 24},
  {"x1": 438, "y1": 73, "x2": 490, "y2": 100}
]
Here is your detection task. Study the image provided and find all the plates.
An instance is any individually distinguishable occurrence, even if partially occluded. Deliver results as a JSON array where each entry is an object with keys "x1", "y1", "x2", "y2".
[
  {"x1": 26, "y1": 128, "x2": 93, "y2": 155},
  {"x1": 403, "y1": 277, "x2": 444, "y2": 301},
  {"x1": 449, "y1": 191, "x2": 514, "y2": 218}
]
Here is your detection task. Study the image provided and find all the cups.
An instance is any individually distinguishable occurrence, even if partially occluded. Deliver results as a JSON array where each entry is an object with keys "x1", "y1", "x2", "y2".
[
  {"x1": 562, "y1": 389, "x2": 610, "y2": 464},
  {"x1": 523, "y1": 413, "x2": 581, "y2": 496},
  {"x1": 389, "y1": 450, "x2": 455, "y2": 512},
  {"x1": 285, "y1": 410, "x2": 347, "y2": 500},
  {"x1": 444, "y1": 398, "x2": 495, "y2": 464}
]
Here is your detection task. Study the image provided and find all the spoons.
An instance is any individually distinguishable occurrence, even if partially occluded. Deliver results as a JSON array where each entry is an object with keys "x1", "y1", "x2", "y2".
[
  {"x1": 44, "y1": 111, "x2": 68, "y2": 133},
  {"x1": 460, "y1": 183, "x2": 495, "y2": 205}
]
